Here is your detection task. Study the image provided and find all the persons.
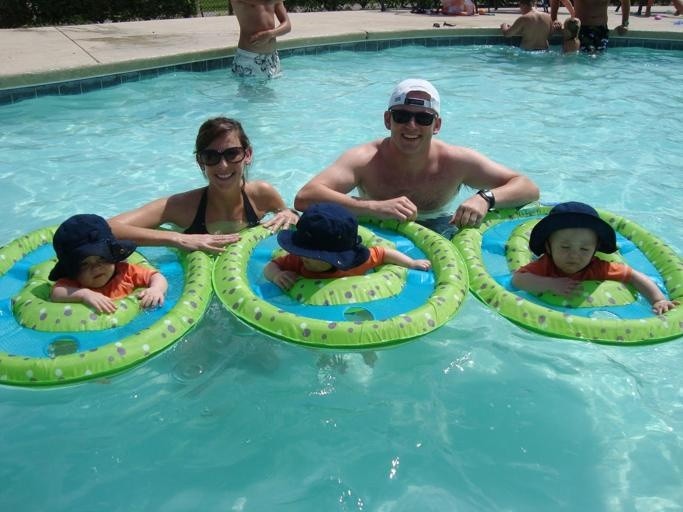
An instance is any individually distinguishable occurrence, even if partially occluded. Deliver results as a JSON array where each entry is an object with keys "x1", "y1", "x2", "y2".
[
  {"x1": 104, "y1": 117, "x2": 300, "y2": 253},
  {"x1": 228, "y1": 1, "x2": 291, "y2": 77},
  {"x1": 49, "y1": 214, "x2": 167, "y2": 312},
  {"x1": 583, "y1": 31, "x2": 595, "y2": 53},
  {"x1": 502, "y1": 0, "x2": 553, "y2": 50},
  {"x1": 295, "y1": 78, "x2": 538, "y2": 226},
  {"x1": 546, "y1": 1, "x2": 628, "y2": 52},
  {"x1": 544, "y1": 0, "x2": 548, "y2": 12},
  {"x1": 264, "y1": 203, "x2": 432, "y2": 295},
  {"x1": 441, "y1": 0, "x2": 476, "y2": 14},
  {"x1": 512, "y1": 200, "x2": 680, "y2": 316}
]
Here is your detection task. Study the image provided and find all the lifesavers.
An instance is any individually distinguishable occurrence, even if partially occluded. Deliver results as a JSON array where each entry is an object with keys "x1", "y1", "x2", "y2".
[
  {"x1": 213, "y1": 220, "x2": 467, "y2": 349},
  {"x1": 450, "y1": 204, "x2": 683, "y2": 343},
  {"x1": 0, "y1": 224, "x2": 212, "y2": 387}
]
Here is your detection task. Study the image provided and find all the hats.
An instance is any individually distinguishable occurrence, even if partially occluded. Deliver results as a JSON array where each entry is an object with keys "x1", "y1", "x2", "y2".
[
  {"x1": 277, "y1": 201, "x2": 370, "y2": 271},
  {"x1": 387, "y1": 78, "x2": 440, "y2": 116},
  {"x1": 530, "y1": 201, "x2": 619, "y2": 257},
  {"x1": 48, "y1": 214, "x2": 138, "y2": 280}
]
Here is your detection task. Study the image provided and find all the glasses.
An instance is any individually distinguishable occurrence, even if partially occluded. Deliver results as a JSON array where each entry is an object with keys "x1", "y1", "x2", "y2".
[
  {"x1": 196, "y1": 146, "x2": 249, "y2": 166},
  {"x1": 391, "y1": 109, "x2": 433, "y2": 126}
]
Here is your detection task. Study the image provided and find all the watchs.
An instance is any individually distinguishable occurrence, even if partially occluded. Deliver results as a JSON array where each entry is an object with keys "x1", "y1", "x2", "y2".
[{"x1": 477, "y1": 188, "x2": 496, "y2": 211}]
